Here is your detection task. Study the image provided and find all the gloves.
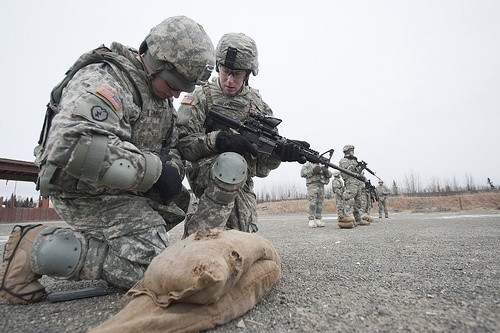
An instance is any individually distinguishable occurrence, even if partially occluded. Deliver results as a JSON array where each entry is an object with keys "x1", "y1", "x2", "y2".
[
  {"x1": 322, "y1": 166, "x2": 328, "y2": 176},
  {"x1": 278, "y1": 140, "x2": 310, "y2": 164},
  {"x1": 213, "y1": 131, "x2": 258, "y2": 157},
  {"x1": 313, "y1": 165, "x2": 322, "y2": 173},
  {"x1": 353, "y1": 157, "x2": 357, "y2": 161},
  {"x1": 361, "y1": 164, "x2": 366, "y2": 169},
  {"x1": 150, "y1": 161, "x2": 183, "y2": 204}
]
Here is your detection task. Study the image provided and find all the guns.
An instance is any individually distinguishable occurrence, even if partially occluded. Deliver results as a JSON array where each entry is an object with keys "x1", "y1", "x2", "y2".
[
  {"x1": 355, "y1": 160, "x2": 380, "y2": 180},
  {"x1": 203, "y1": 111, "x2": 366, "y2": 183}
]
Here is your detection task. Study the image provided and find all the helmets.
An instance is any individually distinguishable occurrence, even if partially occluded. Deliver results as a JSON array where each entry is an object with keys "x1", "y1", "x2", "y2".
[
  {"x1": 343, "y1": 145, "x2": 355, "y2": 153},
  {"x1": 145, "y1": 15, "x2": 216, "y2": 84},
  {"x1": 333, "y1": 171, "x2": 341, "y2": 177},
  {"x1": 378, "y1": 180, "x2": 384, "y2": 183},
  {"x1": 214, "y1": 32, "x2": 259, "y2": 76}
]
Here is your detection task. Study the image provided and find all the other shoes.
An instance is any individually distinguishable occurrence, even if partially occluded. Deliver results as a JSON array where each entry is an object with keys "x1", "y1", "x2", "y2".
[
  {"x1": 309, "y1": 219, "x2": 317, "y2": 228},
  {"x1": 316, "y1": 219, "x2": 326, "y2": 226},
  {"x1": 385, "y1": 213, "x2": 388, "y2": 218},
  {"x1": 0, "y1": 222, "x2": 47, "y2": 304},
  {"x1": 379, "y1": 214, "x2": 382, "y2": 218},
  {"x1": 355, "y1": 219, "x2": 370, "y2": 225}
]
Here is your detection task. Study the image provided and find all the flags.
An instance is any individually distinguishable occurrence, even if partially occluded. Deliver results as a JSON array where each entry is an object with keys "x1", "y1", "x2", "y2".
[{"x1": 5, "y1": 180, "x2": 8, "y2": 185}]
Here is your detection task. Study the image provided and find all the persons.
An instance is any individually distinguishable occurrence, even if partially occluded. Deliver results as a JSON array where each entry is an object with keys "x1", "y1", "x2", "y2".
[
  {"x1": 332, "y1": 144, "x2": 392, "y2": 226},
  {"x1": 301, "y1": 160, "x2": 332, "y2": 228},
  {"x1": 176, "y1": 32, "x2": 310, "y2": 239},
  {"x1": 0, "y1": 15, "x2": 217, "y2": 306}
]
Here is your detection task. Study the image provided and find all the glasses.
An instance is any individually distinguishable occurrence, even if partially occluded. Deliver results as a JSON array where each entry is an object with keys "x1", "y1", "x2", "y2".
[{"x1": 219, "y1": 64, "x2": 247, "y2": 78}]
